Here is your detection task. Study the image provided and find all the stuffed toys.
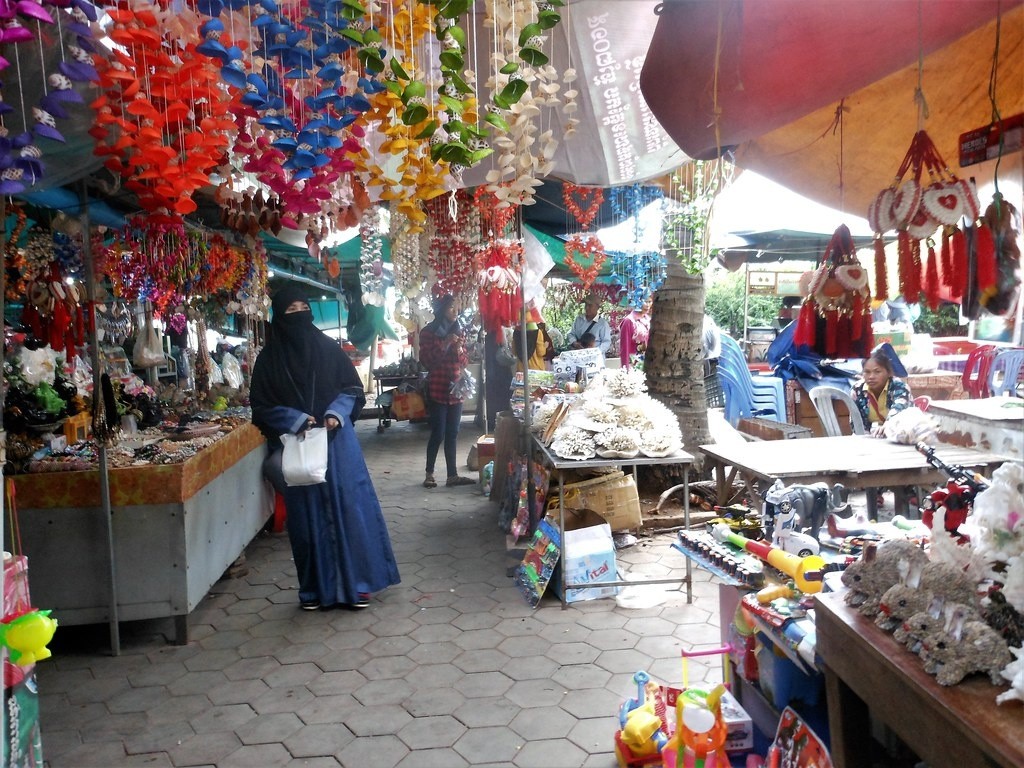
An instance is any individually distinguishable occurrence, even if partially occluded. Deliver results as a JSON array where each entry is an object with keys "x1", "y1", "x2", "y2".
[{"x1": 841, "y1": 539, "x2": 1024, "y2": 706}]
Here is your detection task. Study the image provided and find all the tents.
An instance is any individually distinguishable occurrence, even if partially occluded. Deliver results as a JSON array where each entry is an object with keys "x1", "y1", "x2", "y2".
[{"x1": 0, "y1": 0, "x2": 1024, "y2": 658}]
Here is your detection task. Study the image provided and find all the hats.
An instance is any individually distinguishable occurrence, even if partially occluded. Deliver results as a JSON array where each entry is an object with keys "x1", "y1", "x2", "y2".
[{"x1": 580, "y1": 295, "x2": 601, "y2": 305}]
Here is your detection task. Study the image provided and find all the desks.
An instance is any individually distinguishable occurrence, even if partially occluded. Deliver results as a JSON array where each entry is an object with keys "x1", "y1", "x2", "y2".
[
  {"x1": 928, "y1": 397, "x2": 1024, "y2": 427},
  {"x1": 810, "y1": 585, "x2": 1024, "y2": 768},
  {"x1": 698, "y1": 432, "x2": 1010, "y2": 542}
]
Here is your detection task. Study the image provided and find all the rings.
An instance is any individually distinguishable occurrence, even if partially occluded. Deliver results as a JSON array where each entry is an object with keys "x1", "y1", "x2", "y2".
[
  {"x1": 308, "y1": 421, "x2": 312, "y2": 425},
  {"x1": 334, "y1": 422, "x2": 338, "y2": 426}
]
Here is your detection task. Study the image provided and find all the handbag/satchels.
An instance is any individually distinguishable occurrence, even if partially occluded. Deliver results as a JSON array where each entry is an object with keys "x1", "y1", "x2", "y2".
[
  {"x1": 391, "y1": 384, "x2": 427, "y2": 420},
  {"x1": 280, "y1": 427, "x2": 330, "y2": 486},
  {"x1": 133, "y1": 320, "x2": 167, "y2": 367}
]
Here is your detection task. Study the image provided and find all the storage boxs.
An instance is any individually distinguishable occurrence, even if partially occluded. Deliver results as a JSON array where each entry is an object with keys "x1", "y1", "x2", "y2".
[
  {"x1": 547, "y1": 463, "x2": 646, "y2": 605},
  {"x1": 683, "y1": 627, "x2": 831, "y2": 768},
  {"x1": 0, "y1": 554, "x2": 44, "y2": 768},
  {"x1": 477, "y1": 432, "x2": 496, "y2": 487}
]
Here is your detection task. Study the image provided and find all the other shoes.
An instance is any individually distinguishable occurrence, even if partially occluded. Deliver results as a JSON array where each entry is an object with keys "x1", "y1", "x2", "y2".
[
  {"x1": 302, "y1": 602, "x2": 320, "y2": 610},
  {"x1": 907, "y1": 497, "x2": 918, "y2": 505},
  {"x1": 877, "y1": 495, "x2": 884, "y2": 507},
  {"x1": 446, "y1": 476, "x2": 476, "y2": 485},
  {"x1": 423, "y1": 478, "x2": 437, "y2": 487},
  {"x1": 351, "y1": 594, "x2": 370, "y2": 607}
]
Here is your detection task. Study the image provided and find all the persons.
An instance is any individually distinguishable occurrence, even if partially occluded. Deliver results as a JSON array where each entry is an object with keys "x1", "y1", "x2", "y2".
[
  {"x1": 847, "y1": 349, "x2": 917, "y2": 508},
  {"x1": 512, "y1": 323, "x2": 550, "y2": 371},
  {"x1": 238, "y1": 277, "x2": 401, "y2": 609},
  {"x1": 581, "y1": 333, "x2": 596, "y2": 348},
  {"x1": 567, "y1": 294, "x2": 610, "y2": 362},
  {"x1": 418, "y1": 295, "x2": 475, "y2": 486},
  {"x1": 702, "y1": 313, "x2": 721, "y2": 376},
  {"x1": 619, "y1": 290, "x2": 655, "y2": 369}
]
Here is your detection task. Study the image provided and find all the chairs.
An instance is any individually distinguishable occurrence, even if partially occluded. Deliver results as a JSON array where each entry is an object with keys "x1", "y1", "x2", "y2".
[
  {"x1": 809, "y1": 386, "x2": 865, "y2": 437},
  {"x1": 964, "y1": 345, "x2": 1024, "y2": 396},
  {"x1": 714, "y1": 334, "x2": 787, "y2": 431}
]
